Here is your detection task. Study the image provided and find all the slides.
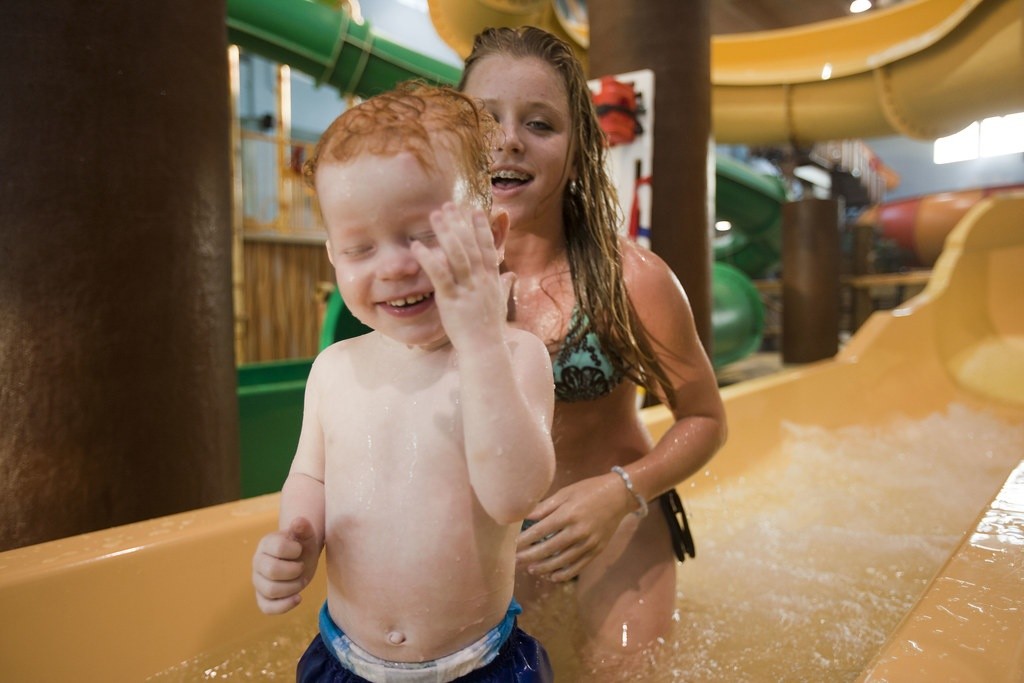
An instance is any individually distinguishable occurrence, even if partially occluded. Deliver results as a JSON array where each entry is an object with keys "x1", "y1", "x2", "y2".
[{"x1": 0, "y1": 0, "x2": 1024, "y2": 683}]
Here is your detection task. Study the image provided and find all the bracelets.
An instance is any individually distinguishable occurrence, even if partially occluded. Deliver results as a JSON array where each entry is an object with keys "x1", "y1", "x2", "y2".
[{"x1": 612, "y1": 465, "x2": 649, "y2": 518}]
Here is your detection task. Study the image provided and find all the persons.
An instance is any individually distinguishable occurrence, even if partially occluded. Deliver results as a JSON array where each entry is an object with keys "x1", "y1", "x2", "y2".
[
  {"x1": 457, "y1": 24, "x2": 727, "y2": 652},
  {"x1": 253, "y1": 79, "x2": 555, "y2": 683}
]
